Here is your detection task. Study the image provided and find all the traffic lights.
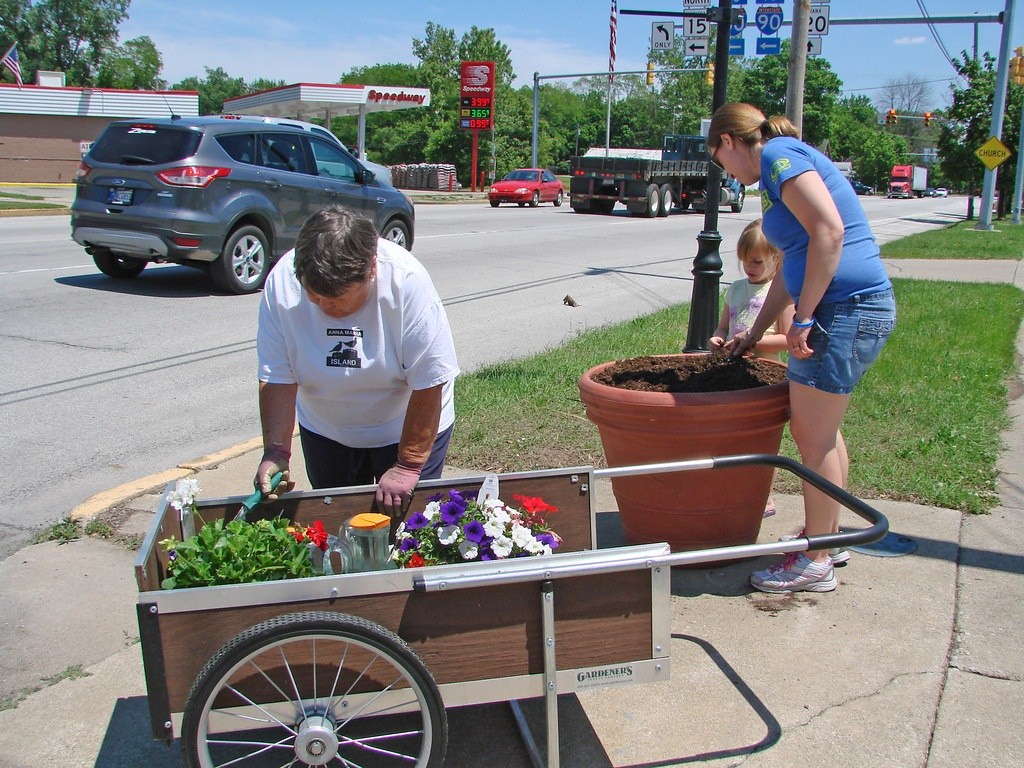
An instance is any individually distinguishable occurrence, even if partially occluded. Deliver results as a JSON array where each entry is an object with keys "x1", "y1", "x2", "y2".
[
  {"x1": 923, "y1": 112, "x2": 931, "y2": 127},
  {"x1": 1010, "y1": 46, "x2": 1024, "y2": 88},
  {"x1": 885, "y1": 110, "x2": 897, "y2": 125}
]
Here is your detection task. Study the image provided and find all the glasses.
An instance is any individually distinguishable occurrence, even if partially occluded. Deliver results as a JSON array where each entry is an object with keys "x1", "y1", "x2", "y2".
[{"x1": 710, "y1": 138, "x2": 725, "y2": 172}]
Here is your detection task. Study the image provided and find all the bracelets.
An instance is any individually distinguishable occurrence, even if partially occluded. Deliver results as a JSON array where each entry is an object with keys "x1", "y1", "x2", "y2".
[
  {"x1": 792, "y1": 313, "x2": 815, "y2": 328},
  {"x1": 263, "y1": 446, "x2": 292, "y2": 456},
  {"x1": 745, "y1": 328, "x2": 760, "y2": 344}
]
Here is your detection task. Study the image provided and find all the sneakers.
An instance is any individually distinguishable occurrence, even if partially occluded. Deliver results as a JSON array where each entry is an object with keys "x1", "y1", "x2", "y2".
[
  {"x1": 777, "y1": 526, "x2": 850, "y2": 564},
  {"x1": 750, "y1": 552, "x2": 837, "y2": 594}
]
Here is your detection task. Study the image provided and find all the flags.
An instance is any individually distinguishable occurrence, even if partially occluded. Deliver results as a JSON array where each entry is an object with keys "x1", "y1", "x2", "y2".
[{"x1": 1, "y1": 46, "x2": 23, "y2": 91}]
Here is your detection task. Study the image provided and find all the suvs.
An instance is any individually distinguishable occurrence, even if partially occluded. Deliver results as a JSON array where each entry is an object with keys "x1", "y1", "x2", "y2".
[
  {"x1": 200, "y1": 111, "x2": 395, "y2": 188},
  {"x1": 67, "y1": 114, "x2": 416, "y2": 296}
]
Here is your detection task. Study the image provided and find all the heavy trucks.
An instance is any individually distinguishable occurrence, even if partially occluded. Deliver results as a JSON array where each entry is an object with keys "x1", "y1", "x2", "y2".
[
  {"x1": 885, "y1": 164, "x2": 927, "y2": 199},
  {"x1": 565, "y1": 134, "x2": 747, "y2": 219}
]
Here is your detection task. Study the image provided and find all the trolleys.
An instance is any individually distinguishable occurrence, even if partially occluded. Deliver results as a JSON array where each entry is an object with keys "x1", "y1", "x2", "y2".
[{"x1": 133, "y1": 450, "x2": 894, "y2": 768}]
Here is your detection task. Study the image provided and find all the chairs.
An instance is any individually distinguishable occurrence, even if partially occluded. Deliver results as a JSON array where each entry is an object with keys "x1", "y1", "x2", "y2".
[{"x1": 233, "y1": 139, "x2": 291, "y2": 170}]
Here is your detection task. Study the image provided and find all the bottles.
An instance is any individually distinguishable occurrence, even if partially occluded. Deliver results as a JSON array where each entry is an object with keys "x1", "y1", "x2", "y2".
[{"x1": 324, "y1": 518, "x2": 399, "y2": 574}]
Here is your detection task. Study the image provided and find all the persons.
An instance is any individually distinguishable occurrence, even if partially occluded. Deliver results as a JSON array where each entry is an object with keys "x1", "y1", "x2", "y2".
[
  {"x1": 253, "y1": 203, "x2": 455, "y2": 519},
  {"x1": 706, "y1": 101, "x2": 897, "y2": 595},
  {"x1": 707, "y1": 219, "x2": 797, "y2": 364}
]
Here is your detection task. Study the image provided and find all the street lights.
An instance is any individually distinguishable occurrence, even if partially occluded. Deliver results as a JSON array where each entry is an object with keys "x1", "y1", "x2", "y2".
[{"x1": 672, "y1": 104, "x2": 683, "y2": 135}]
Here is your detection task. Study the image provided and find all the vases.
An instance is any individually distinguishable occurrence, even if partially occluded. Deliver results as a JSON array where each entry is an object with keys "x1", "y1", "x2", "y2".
[{"x1": 580, "y1": 351, "x2": 790, "y2": 570}]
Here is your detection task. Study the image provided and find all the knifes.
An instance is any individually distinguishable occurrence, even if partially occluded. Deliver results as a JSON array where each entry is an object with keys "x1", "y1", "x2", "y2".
[{"x1": 233, "y1": 471, "x2": 284, "y2": 521}]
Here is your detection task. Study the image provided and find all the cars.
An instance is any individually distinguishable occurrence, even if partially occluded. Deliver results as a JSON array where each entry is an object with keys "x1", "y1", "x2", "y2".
[
  {"x1": 849, "y1": 180, "x2": 875, "y2": 196},
  {"x1": 923, "y1": 186, "x2": 948, "y2": 198},
  {"x1": 487, "y1": 168, "x2": 564, "y2": 207}
]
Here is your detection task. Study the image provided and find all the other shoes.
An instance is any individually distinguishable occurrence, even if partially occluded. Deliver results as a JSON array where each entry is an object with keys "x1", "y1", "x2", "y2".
[{"x1": 763, "y1": 501, "x2": 775, "y2": 517}]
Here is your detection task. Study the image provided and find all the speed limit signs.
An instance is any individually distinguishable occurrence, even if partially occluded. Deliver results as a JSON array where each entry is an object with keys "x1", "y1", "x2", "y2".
[
  {"x1": 681, "y1": 8, "x2": 712, "y2": 38},
  {"x1": 807, "y1": 5, "x2": 830, "y2": 36}
]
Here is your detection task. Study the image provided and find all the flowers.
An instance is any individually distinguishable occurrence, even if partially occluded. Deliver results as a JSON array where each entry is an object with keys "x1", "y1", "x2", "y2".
[
  {"x1": 156, "y1": 475, "x2": 331, "y2": 589},
  {"x1": 392, "y1": 476, "x2": 561, "y2": 572}
]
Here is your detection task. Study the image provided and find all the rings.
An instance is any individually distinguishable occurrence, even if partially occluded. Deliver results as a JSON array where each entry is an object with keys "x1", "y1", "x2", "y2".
[{"x1": 793, "y1": 346, "x2": 799, "y2": 352}]
